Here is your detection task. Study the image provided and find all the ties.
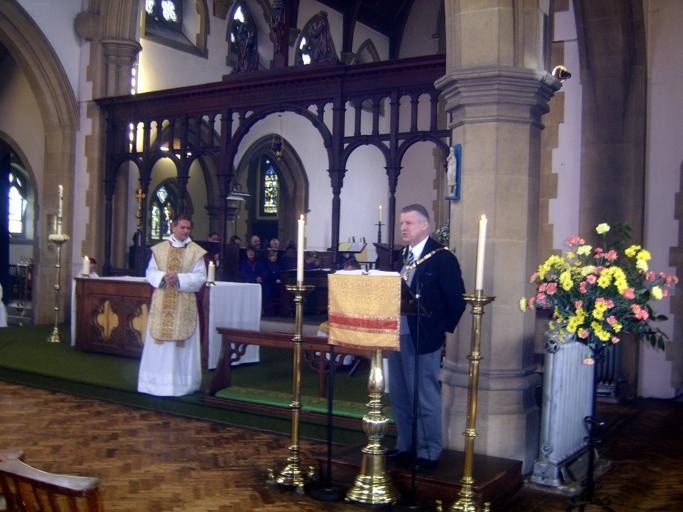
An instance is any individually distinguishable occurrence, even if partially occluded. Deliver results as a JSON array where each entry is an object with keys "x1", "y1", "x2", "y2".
[{"x1": 407, "y1": 251, "x2": 413, "y2": 265}]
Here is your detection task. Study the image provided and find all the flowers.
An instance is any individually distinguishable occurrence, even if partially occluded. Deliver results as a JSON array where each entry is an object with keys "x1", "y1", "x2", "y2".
[{"x1": 520, "y1": 223, "x2": 679, "y2": 365}]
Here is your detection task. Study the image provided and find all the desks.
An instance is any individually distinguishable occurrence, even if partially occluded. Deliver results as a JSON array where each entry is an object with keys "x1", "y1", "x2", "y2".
[{"x1": 204, "y1": 326, "x2": 389, "y2": 398}]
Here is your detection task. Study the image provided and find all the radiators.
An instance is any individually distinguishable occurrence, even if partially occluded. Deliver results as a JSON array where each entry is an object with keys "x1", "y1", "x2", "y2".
[{"x1": 531, "y1": 342, "x2": 599, "y2": 487}]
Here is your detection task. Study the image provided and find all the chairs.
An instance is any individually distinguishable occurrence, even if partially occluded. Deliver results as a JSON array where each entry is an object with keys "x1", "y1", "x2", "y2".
[{"x1": 0, "y1": 458, "x2": 102, "y2": 512}]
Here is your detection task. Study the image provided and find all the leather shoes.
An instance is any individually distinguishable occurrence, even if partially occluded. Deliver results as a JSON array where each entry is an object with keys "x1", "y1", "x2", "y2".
[{"x1": 385, "y1": 448, "x2": 432, "y2": 474}]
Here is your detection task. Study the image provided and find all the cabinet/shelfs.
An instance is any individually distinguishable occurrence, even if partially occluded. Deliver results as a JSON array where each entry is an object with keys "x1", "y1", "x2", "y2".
[{"x1": 76, "y1": 277, "x2": 262, "y2": 367}]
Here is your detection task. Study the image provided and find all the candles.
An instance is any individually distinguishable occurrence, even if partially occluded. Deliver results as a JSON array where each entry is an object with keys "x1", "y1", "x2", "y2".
[
  {"x1": 297, "y1": 214, "x2": 305, "y2": 281},
  {"x1": 379, "y1": 205, "x2": 382, "y2": 221},
  {"x1": 476, "y1": 214, "x2": 488, "y2": 289},
  {"x1": 56, "y1": 185, "x2": 63, "y2": 235},
  {"x1": 207, "y1": 260, "x2": 215, "y2": 281},
  {"x1": 82, "y1": 255, "x2": 90, "y2": 274}
]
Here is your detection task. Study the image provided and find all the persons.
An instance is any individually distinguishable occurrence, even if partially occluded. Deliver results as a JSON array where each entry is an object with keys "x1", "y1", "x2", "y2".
[
  {"x1": 76, "y1": 257, "x2": 99, "y2": 278},
  {"x1": 134, "y1": 213, "x2": 207, "y2": 398},
  {"x1": 385, "y1": 203, "x2": 468, "y2": 471},
  {"x1": 204, "y1": 231, "x2": 356, "y2": 316}
]
